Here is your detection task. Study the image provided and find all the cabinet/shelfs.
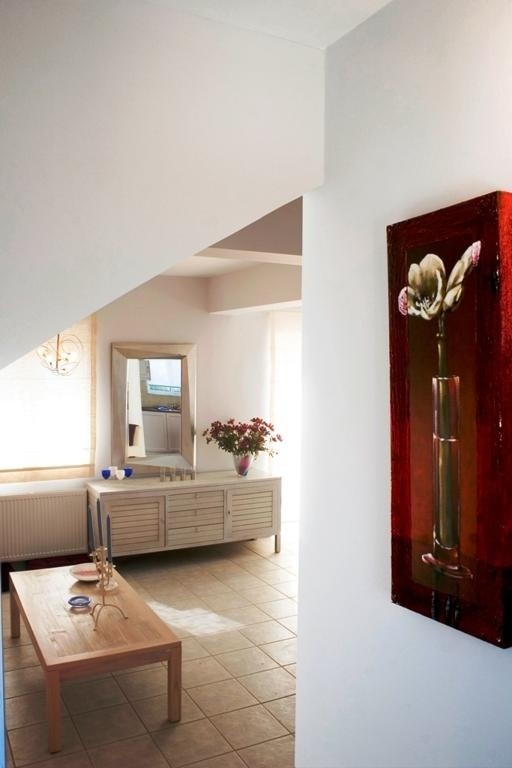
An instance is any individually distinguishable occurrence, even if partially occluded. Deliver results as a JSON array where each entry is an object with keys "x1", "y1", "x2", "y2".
[
  {"x1": 87, "y1": 469, "x2": 282, "y2": 558},
  {"x1": 142, "y1": 410, "x2": 183, "y2": 454}
]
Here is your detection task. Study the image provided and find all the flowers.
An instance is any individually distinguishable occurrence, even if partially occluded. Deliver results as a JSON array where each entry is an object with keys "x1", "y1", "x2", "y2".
[
  {"x1": 399, "y1": 239, "x2": 482, "y2": 549},
  {"x1": 203, "y1": 417, "x2": 284, "y2": 461}
]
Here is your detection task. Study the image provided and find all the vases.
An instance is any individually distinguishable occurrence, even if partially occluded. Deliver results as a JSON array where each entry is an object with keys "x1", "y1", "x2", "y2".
[
  {"x1": 232, "y1": 453, "x2": 252, "y2": 476},
  {"x1": 418, "y1": 375, "x2": 476, "y2": 580}
]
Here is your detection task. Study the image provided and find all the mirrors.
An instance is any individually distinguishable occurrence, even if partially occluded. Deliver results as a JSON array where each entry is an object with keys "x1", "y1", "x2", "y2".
[{"x1": 110, "y1": 342, "x2": 196, "y2": 473}]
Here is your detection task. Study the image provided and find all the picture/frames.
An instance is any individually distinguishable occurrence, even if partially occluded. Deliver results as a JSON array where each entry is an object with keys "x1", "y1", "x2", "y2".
[{"x1": 387, "y1": 190, "x2": 512, "y2": 649}]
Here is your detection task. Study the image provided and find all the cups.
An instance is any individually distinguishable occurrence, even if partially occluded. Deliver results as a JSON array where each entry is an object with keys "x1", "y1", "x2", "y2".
[
  {"x1": 124, "y1": 468, "x2": 133, "y2": 478},
  {"x1": 115, "y1": 470, "x2": 125, "y2": 481},
  {"x1": 102, "y1": 470, "x2": 111, "y2": 480},
  {"x1": 109, "y1": 466, "x2": 118, "y2": 476}
]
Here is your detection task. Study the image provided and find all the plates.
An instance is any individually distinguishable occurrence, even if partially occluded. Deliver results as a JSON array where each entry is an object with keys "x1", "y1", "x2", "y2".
[{"x1": 70, "y1": 563, "x2": 113, "y2": 581}]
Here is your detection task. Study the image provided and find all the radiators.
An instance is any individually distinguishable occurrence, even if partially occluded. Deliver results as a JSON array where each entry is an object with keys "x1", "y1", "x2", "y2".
[{"x1": 0, "y1": 488, "x2": 88, "y2": 562}]
